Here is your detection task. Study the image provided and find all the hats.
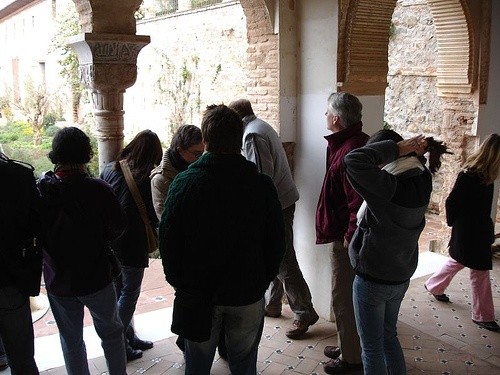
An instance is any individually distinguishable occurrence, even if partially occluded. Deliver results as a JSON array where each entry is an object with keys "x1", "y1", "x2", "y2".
[{"x1": 327, "y1": 92, "x2": 363, "y2": 129}]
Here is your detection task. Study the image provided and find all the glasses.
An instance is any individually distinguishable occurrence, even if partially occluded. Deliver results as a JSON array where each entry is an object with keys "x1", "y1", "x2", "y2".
[{"x1": 186, "y1": 149, "x2": 203, "y2": 158}]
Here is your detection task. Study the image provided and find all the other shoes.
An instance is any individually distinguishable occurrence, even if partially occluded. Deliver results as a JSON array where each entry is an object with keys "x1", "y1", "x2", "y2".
[
  {"x1": 125, "y1": 335, "x2": 153, "y2": 359},
  {"x1": 0, "y1": 363, "x2": 8, "y2": 370},
  {"x1": 424, "y1": 284, "x2": 449, "y2": 301},
  {"x1": 472, "y1": 319, "x2": 500, "y2": 332}
]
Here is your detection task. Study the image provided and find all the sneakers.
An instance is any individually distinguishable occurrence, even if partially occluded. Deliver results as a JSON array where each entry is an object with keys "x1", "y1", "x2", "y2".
[
  {"x1": 264, "y1": 311, "x2": 281, "y2": 317},
  {"x1": 324, "y1": 346, "x2": 342, "y2": 359},
  {"x1": 324, "y1": 358, "x2": 362, "y2": 373},
  {"x1": 285, "y1": 312, "x2": 319, "y2": 335}
]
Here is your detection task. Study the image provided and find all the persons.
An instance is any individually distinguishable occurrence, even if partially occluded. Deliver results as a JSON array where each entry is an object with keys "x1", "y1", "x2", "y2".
[
  {"x1": 150, "y1": 125, "x2": 205, "y2": 221},
  {"x1": 231, "y1": 98, "x2": 319, "y2": 335},
  {"x1": 159, "y1": 104, "x2": 286, "y2": 375},
  {"x1": 0, "y1": 152, "x2": 42, "y2": 375},
  {"x1": 100, "y1": 129, "x2": 163, "y2": 361},
  {"x1": 424, "y1": 133, "x2": 500, "y2": 331},
  {"x1": 343, "y1": 130, "x2": 453, "y2": 375},
  {"x1": 316, "y1": 92, "x2": 371, "y2": 375},
  {"x1": 35, "y1": 126, "x2": 126, "y2": 375}
]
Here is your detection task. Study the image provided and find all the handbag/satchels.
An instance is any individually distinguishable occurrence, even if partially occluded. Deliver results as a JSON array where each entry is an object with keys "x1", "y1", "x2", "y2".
[{"x1": 147, "y1": 224, "x2": 158, "y2": 253}]
